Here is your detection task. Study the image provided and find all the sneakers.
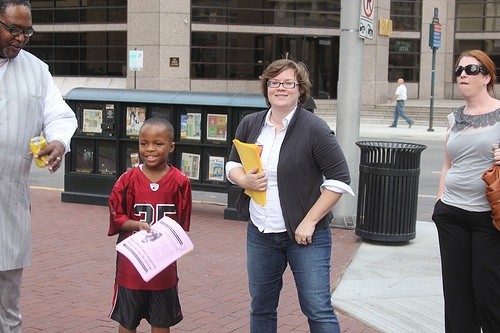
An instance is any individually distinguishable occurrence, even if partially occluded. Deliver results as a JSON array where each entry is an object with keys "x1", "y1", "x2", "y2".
[
  {"x1": 409, "y1": 121, "x2": 414, "y2": 128},
  {"x1": 389, "y1": 124, "x2": 396, "y2": 127}
]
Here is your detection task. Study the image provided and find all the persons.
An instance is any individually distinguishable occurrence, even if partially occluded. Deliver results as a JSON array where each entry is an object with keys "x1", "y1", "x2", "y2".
[
  {"x1": 431, "y1": 50, "x2": 500, "y2": 333},
  {"x1": 389, "y1": 78, "x2": 414, "y2": 128},
  {"x1": 226, "y1": 59, "x2": 354, "y2": 333},
  {"x1": 108, "y1": 116, "x2": 192, "y2": 333},
  {"x1": 0, "y1": 0, "x2": 78, "y2": 333}
]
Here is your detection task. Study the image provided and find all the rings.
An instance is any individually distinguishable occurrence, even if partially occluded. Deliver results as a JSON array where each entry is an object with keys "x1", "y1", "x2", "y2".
[
  {"x1": 302, "y1": 240, "x2": 306, "y2": 242},
  {"x1": 56, "y1": 156, "x2": 61, "y2": 161}
]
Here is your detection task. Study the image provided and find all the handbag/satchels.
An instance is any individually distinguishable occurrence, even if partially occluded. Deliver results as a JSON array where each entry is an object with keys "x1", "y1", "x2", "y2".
[{"x1": 480, "y1": 141, "x2": 500, "y2": 231}]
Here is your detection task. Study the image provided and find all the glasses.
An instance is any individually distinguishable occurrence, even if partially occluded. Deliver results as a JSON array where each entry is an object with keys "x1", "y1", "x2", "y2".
[
  {"x1": 0, "y1": 20, "x2": 36, "y2": 38},
  {"x1": 452, "y1": 64, "x2": 488, "y2": 75},
  {"x1": 266, "y1": 80, "x2": 300, "y2": 89}
]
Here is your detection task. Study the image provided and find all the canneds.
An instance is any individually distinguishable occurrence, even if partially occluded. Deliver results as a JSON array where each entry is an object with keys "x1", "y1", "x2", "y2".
[{"x1": 29, "y1": 136, "x2": 52, "y2": 168}]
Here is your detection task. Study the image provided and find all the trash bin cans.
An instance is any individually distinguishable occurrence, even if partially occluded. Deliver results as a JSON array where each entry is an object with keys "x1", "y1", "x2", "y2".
[{"x1": 355, "y1": 141, "x2": 428, "y2": 247}]
